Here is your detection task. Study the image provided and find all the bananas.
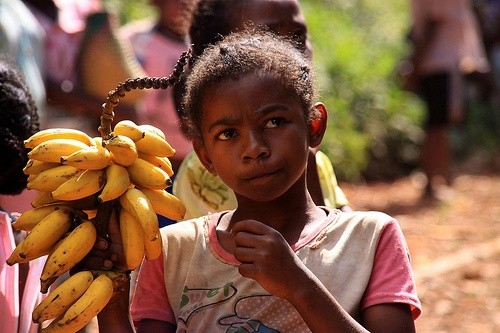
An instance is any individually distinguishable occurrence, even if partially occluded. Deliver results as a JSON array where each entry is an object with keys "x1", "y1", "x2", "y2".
[{"x1": 5, "y1": 120, "x2": 186, "y2": 333}]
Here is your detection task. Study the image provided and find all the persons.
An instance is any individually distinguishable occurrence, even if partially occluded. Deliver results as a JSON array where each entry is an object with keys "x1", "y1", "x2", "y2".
[
  {"x1": 402, "y1": 0, "x2": 491, "y2": 202},
  {"x1": 0, "y1": 1, "x2": 352, "y2": 222},
  {"x1": 74, "y1": 26, "x2": 421, "y2": 333}
]
